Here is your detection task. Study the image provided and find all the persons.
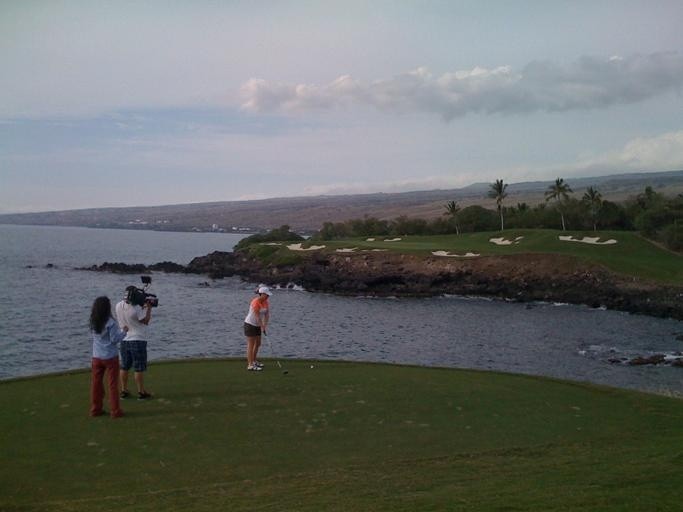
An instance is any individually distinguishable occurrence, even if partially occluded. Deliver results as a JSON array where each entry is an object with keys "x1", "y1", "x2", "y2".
[
  {"x1": 115, "y1": 285, "x2": 152, "y2": 401},
  {"x1": 85, "y1": 295, "x2": 129, "y2": 418},
  {"x1": 243, "y1": 286, "x2": 274, "y2": 371}
]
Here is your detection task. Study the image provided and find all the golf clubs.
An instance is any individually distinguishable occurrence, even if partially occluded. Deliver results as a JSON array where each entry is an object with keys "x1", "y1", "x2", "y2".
[{"x1": 264, "y1": 330, "x2": 288, "y2": 374}]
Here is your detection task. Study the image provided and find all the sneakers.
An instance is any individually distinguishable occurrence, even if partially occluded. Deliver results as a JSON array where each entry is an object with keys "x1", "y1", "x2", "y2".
[
  {"x1": 119, "y1": 389, "x2": 132, "y2": 400},
  {"x1": 248, "y1": 360, "x2": 264, "y2": 371},
  {"x1": 137, "y1": 390, "x2": 154, "y2": 400}
]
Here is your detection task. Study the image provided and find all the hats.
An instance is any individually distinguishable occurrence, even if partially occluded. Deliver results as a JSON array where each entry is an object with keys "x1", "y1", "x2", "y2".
[{"x1": 259, "y1": 287, "x2": 273, "y2": 295}]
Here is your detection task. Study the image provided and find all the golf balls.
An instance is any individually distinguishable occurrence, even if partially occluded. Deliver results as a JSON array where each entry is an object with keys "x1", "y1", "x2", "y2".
[{"x1": 310, "y1": 365, "x2": 314, "y2": 368}]
